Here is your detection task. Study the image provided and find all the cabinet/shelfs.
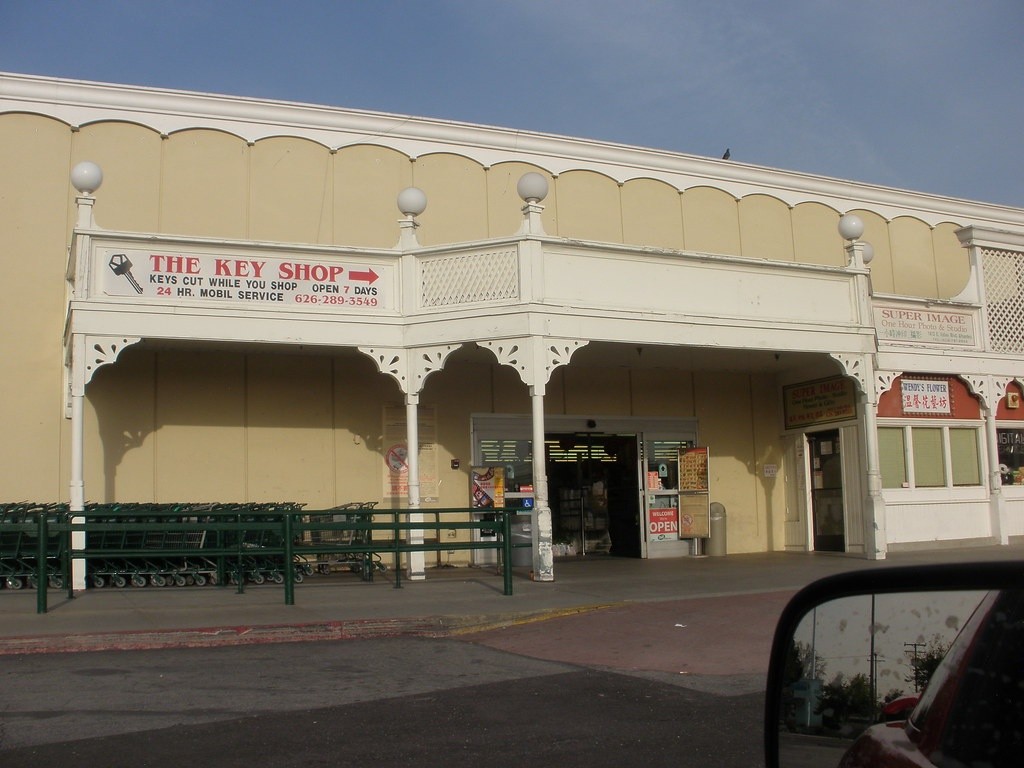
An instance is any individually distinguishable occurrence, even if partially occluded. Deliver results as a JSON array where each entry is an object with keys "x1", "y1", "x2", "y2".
[{"x1": 557, "y1": 486, "x2": 609, "y2": 559}]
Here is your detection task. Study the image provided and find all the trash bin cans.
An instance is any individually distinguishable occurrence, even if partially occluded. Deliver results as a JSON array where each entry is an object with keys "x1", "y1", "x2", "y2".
[
  {"x1": 510, "y1": 515, "x2": 533, "y2": 567},
  {"x1": 704, "y1": 501, "x2": 727, "y2": 557}
]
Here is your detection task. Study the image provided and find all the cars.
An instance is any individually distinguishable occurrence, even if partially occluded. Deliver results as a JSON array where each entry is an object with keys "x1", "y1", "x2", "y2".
[{"x1": 838, "y1": 589, "x2": 1024, "y2": 768}]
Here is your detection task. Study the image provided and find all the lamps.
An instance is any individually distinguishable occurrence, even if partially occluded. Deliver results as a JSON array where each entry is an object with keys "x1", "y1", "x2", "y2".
[
  {"x1": 69, "y1": 159, "x2": 103, "y2": 199},
  {"x1": 516, "y1": 171, "x2": 549, "y2": 203},
  {"x1": 837, "y1": 211, "x2": 865, "y2": 242},
  {"x1": 856, "y1": 240, "x2": 875, "y2": 266},
  {"x1": 396, "y1": 185, "x2": 429, "y2": 219}
]
